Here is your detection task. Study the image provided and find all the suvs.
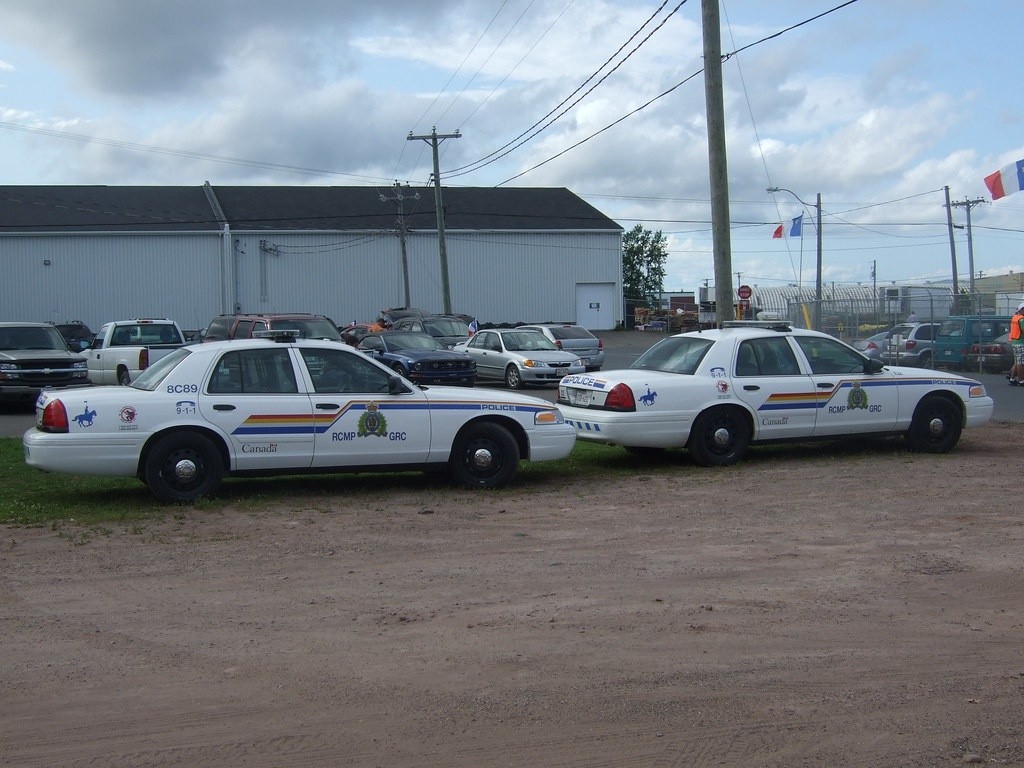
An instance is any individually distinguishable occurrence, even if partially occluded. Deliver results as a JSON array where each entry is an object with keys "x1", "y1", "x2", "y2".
[
  {"x1": 203, "y1": 314, "x2": 347, "y2": 381},
  {"x1": 879, "y1": 320, "x2": 941, "y2": 369},
  {"x1": 387, "y1": 314, "x2": 475, "y2": 350}
]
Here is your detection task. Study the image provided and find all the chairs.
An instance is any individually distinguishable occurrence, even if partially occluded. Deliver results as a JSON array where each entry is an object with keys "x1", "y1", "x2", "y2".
[
  {"x1": 117, "y1": 328, "x2": 130, "y2": 342},
  {"x1": 160, "y1": 327, "x2": 172, "y2": 339},
  {"x1": 742, "y1": 346, "x2": 758, "y2": 375}
]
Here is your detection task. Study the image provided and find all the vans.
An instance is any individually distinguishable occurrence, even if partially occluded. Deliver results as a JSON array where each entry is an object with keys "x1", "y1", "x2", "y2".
[{"x1": 934, "y1": 314, "x2": 1013, "y2": 369}]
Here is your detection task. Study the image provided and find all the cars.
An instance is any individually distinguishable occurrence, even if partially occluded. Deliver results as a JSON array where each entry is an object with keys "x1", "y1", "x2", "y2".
[
  {"x1": 556, "y1": 320, "x2": 993, "y2": 467},
  {"x1": 339, "y1": 324, "x2": 387, "y2": 346},
  {"x1": 514, "y1": 323, "x2": 605, "y2": 372},
  {"x1": 23, "y1": 329, "x2": 577, "y2": 502},
  {"x1": 847, "y1": 331, "x2": 889, "y2": 364},
  {"x1": 65, "y1": 335, "x2": 80, "y2": 349},
  {"x1": 454, "y1": 328, "x2": 585, "y2": 389},
  {"x1": 354, "y1": 332, "x2": 477, "y2": 388},
  {"x1": 972, "y1": 333, "x2": 1014, "y2": 374},
  {"x1": 0, "y1": 322, "x2": 94, "y2": 393}
]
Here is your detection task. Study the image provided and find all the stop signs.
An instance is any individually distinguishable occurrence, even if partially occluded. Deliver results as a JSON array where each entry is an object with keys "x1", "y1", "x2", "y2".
[{"x1": 738, "y1": 285, "x2": 752, "y2": 299}]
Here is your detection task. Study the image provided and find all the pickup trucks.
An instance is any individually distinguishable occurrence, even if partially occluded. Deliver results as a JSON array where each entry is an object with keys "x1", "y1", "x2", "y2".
[{"x1": 77, "y1": 318, "x2": 199, "y2": 387}]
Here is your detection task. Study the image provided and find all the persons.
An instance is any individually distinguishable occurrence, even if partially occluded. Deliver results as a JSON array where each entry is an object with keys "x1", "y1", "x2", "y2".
[
  {"x1": 370, "y1": 318, "x2": 387, "y2": 333},
  {"x1": 1006, "y1": 303, "x2": 1024, "y2": 387},
  {"x1": 907, "y1": 311, "x2": 917, "y2": 323}
]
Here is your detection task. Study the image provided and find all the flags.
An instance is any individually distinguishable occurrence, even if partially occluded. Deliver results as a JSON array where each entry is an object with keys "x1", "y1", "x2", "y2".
[
  {"x1": 790, "y1": 215, "x2": 802, "y2": 237},
  {"x1": 983, "y1": 159, "x2": 1024, "y2": 200},
  {"x1": 773, "y1": 225, "x2": 783, "y2": 238},
  {"x1": 468, "y1": 320, "x2": 477, "y2": 336}
]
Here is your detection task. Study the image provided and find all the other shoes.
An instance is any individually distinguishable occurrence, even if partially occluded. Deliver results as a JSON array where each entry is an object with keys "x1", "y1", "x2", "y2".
[
  {"x1": 1017, "y1": 381, "x2": 1024, "y2": 387},
  {"x1": 1009, "y1": 379, "x2": 1018, "y2": 386},
  {"x1": 1006, "y1": 375, "x2": 1010, "y2": 380}
]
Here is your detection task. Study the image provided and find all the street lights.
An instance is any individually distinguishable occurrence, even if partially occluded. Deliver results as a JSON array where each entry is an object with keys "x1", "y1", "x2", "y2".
[{"x1": 766, "y1": 186, "x2": 821, "y2": 331}]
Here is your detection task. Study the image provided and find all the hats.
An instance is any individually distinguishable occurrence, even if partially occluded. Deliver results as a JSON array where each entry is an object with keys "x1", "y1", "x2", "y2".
[{"x1": 1017, "y1": 304, "x2": 1024, "y2": 312}]
